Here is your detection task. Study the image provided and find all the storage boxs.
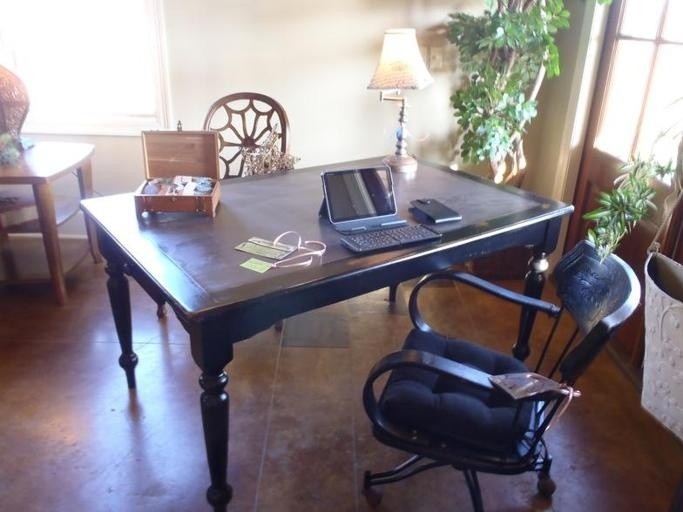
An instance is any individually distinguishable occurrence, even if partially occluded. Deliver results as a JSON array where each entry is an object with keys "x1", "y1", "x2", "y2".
[{"x1": 132, "y1": 130, "x2": 221, "y2": 219}]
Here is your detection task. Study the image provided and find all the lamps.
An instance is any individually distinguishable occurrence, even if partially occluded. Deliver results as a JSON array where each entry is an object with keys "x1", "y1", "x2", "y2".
[{"x1": 367, "y1": 28, "x2": 435, "y2": 175}]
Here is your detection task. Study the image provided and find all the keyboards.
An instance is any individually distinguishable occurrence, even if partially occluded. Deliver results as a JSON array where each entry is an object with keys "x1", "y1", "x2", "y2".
[{"x1": 339, "y1": 224, "x2": 443, "y2": 255}]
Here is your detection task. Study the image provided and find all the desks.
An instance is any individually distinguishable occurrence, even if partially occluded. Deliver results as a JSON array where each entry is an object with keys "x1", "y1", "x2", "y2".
[{"x1": 77, "y1": 154, "x2": 576, "y2": 512}]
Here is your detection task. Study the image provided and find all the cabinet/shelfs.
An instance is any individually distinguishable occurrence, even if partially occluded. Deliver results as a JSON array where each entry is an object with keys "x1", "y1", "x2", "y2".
[{"x1": 0, "y1": 139, "x2": 103, "y2": 306}]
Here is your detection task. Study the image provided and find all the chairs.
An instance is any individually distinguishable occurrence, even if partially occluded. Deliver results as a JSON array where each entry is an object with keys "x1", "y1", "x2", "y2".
[
  {"x1": 361, "y1": 234, "x2": 644, "y2": 512},
  {"x1": 204, "y1": 92, "x2": 288, "y2": 180}
]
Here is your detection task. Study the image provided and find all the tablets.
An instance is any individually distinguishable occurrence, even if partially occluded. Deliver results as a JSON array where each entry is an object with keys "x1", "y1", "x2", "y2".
[{"x1": 321, "y1": 164, "x2": 396, "y2": 225}]
